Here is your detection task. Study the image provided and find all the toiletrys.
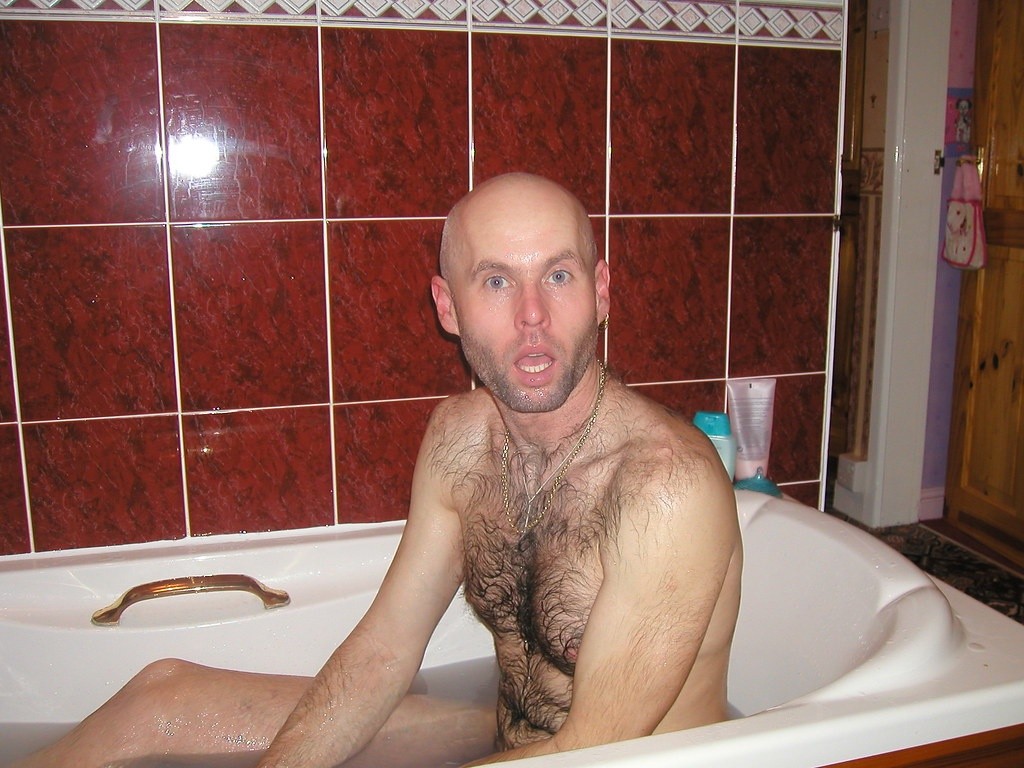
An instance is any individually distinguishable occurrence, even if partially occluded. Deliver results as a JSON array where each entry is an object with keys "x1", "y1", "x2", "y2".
[
  {"x1": 693, "y1": 411, "x2": 736, "y2": 485},
  {"x1": 725, "y1": 378, "x2": 777, "y2": 481}
]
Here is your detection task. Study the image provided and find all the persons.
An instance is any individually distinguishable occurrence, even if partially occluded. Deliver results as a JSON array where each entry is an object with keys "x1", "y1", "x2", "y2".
[{"x1": 4, "y1": 173, "x2": 743, "y2": 768}]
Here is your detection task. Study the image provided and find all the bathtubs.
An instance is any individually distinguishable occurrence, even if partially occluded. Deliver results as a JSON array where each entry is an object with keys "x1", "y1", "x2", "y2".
[{"x1": 0, "y1": 483, "x2": 1024, "y2": 768}]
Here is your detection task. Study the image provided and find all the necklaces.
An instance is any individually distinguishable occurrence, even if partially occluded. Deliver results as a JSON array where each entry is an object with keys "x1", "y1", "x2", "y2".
[
  {"x1": 516, "y1": 420, "x2": 593, "y2": 530},
  {"x1": 503, "y1": 359, "x2": 605, "y2": 535}
]
